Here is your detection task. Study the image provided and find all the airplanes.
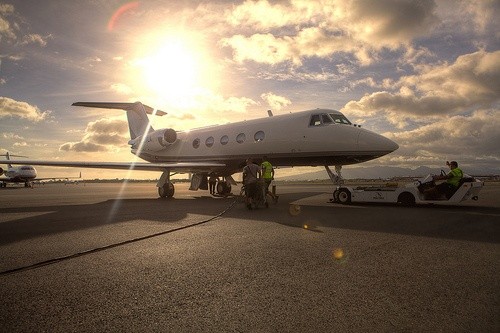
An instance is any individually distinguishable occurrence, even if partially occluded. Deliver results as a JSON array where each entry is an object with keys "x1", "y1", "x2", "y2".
[
  {"x1": 1, "y1": 152, "x2": 81, "y2": 188},
  {"x1": 0, "y1": 101, "x2": 400, "y2": 205}
]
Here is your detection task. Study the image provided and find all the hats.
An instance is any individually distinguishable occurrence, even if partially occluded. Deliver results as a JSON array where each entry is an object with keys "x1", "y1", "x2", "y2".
[{"x1": 447, "y1": 161, "x2": 458, "y2": 167}]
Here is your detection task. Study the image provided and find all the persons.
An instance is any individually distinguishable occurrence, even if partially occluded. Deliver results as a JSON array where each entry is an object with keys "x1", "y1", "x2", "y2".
[
  {"x1": 208, "y1": 171, "x2": 220, "y2": 195},
  {"x1": 432, "y1": 161, "x2": 463, "y2": 200},
  {"x1": 243, "y1": 156, "x2": 279, "y2": 210}
]
